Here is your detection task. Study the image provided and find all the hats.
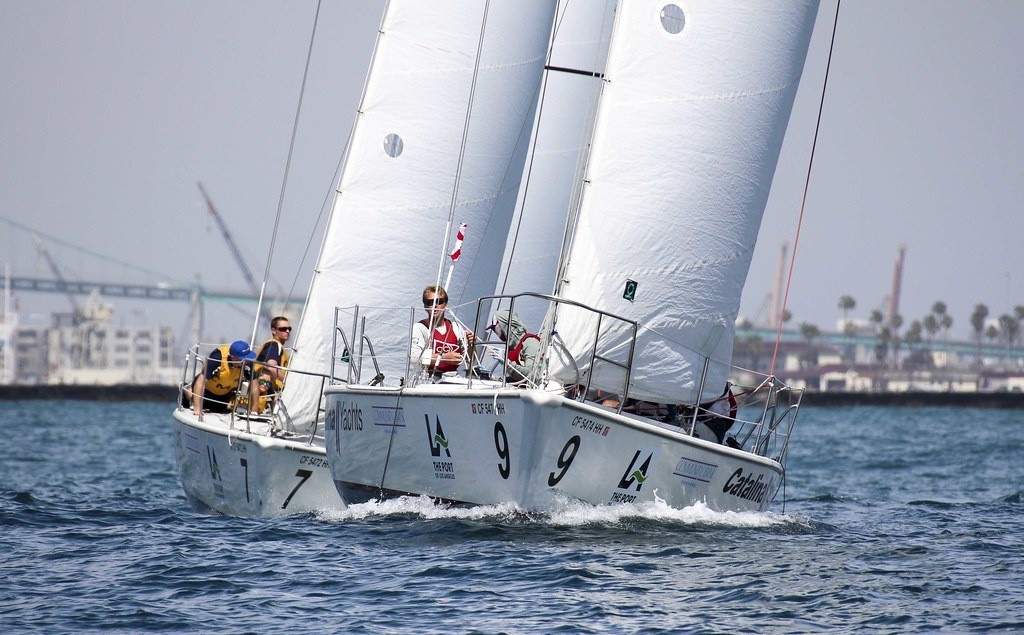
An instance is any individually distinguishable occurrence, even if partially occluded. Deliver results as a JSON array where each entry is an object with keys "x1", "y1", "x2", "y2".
[
  {"x1": 485, "y1": 315, "x2": 500, "y2": 331},
  {"x1": 229, "y1": 341, "x2": 256, "y2": 360}
]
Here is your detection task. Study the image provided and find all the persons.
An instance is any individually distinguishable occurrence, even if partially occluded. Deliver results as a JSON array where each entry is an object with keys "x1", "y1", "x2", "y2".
[
  {"x1": 565, "y1": 379, "x2": 738, "y2": 446},
  {"x1": 183, "y1": 341, "x2": 259, "y2": 417},
  {"x1": 247, "y1": 315, "x2": 292, "y2": 413},
  {"x1": 484, "y1": 309, "x2": 544, "y2": 392},
  {"x1": 409, "y1": 285, "x2": 477, "y2": 383}
]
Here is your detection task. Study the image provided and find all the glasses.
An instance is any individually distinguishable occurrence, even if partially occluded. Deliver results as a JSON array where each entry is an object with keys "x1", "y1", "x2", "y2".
[
  {"x1": 424, "y1": 298, "x2": 445, "y2": 306},
  {"x1": 275, "y1": 326, "x2": 291, "y2": 332}
]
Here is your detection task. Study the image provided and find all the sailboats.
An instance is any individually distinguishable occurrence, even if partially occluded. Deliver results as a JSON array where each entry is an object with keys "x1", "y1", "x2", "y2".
[
  {"x1": 174, "y1": 0, "x2": 558, "y2": 523},
  {"x1": 325, "y1": 0, "x2": 843, "y2": 524}
]
